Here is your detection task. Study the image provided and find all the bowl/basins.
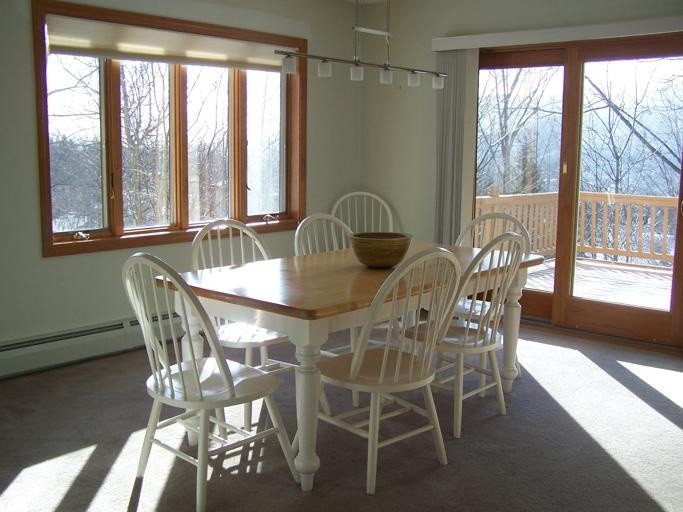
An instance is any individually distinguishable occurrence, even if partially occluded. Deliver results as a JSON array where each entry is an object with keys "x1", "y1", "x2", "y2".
[{"x1": 349, "y1": 231, "x2": 410, "y2": 269}]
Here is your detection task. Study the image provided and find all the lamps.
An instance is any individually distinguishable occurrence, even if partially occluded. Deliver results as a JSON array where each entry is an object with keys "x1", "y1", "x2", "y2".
[
  {"x1": 431, "y1": 75, "x2": 446, "y2": 92},
  {"x1": 283, "y1": 56, "x2": 298, "y2": 76},
  {"x1": 381, "y1": 1, "x2": 393, "y2": 86},
  {"x1": 407, "y1": 72, "x2": 422, "y2": 88},
  {"x1": 318, "y1": 60, "x2": 333, "y2": 80},
  {"x1": 350, "y1": 2, "x2": 364, "y2": 84}
]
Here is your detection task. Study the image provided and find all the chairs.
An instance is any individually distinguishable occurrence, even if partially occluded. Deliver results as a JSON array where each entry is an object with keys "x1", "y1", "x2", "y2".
[
  {"x1": 191, "y1": 220, "x2": 333, "y2": 431},
  {"x1": 331, "y1": 188, "x2": 397, "y2": 251},
  {"x1": 293, "y1": 214, "x2": 386, "y2": 409},
  {"x1": 122, "y1": 252, "x2": 302, "y2": 507},
  {"x1": 435, "y1": 212, "x2": 533, "y2": 399},
  {"x1": 288, "y1": 246, "x2": 463, "y2": 494},
  {"x1": 401, "y1": 231, "x2": 526, "y2": 438}
]
